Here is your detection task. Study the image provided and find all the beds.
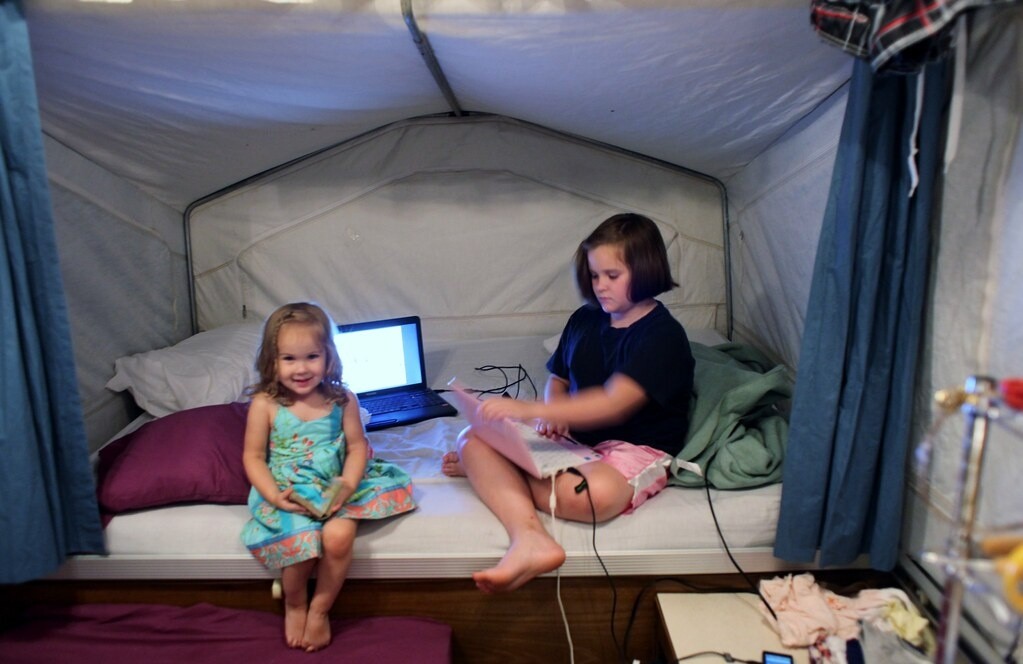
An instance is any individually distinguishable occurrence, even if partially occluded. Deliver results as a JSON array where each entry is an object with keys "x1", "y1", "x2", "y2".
[{"x1": 42, "y1": 333, "x2": 872, "y2": 579}]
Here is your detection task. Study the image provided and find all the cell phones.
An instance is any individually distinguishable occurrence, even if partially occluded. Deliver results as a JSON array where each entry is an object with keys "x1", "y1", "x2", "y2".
[{"x1": 761, "y1": 651, "x2": 794, "y2": 664}]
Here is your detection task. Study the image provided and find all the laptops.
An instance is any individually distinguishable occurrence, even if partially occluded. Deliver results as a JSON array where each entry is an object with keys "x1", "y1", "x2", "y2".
[
  {"x1": 330, "y1": 315, "x2": 459, "y2": 431},
  {"x1": 450, "y1": 384, "x2": 604, "y2": 478}
]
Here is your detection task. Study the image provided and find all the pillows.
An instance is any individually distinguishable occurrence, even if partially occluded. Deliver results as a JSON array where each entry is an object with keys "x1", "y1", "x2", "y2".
[
  {"x1": 104, "y1": 315, "x2": 267, "y2": 419},
  {"x1": 97, "y1": 402, "x2": 252, "y2": 511}
]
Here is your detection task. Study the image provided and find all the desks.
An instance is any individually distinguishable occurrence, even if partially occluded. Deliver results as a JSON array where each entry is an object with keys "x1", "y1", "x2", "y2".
[{"x1": 655, "y1": 592, "x2": 811, "y2": 664}]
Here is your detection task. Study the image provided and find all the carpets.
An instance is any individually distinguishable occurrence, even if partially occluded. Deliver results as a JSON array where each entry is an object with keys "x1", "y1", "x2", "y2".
[{"x1": 0, "y1": 602, "x2": 454, "y2": 664}]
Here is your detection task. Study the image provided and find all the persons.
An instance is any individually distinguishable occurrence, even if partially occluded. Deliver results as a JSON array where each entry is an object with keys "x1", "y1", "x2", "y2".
[
  {"x1": 240, "y1": 303, "x2": 418, "y2": 651},
  {"x1": 441, "y1": 213, "x2": 698, "y2": 596}
]
